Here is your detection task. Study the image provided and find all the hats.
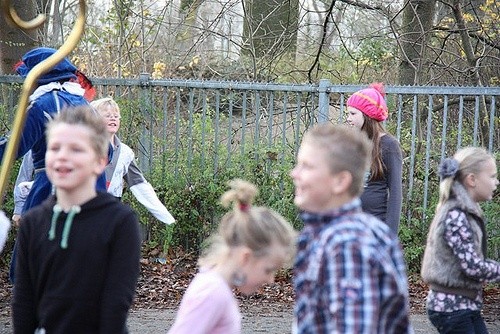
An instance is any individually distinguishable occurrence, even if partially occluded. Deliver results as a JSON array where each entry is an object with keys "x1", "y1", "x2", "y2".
[
  {"x1": 15, "y1": 47, "x2": 77, "y2": 84},
  {"x1": 347, "y1": 82, "x2": 388, "y2": 122}
]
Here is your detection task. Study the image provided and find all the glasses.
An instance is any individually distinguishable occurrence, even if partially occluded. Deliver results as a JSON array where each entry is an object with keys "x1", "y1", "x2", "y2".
[{"x1": 103, "y1": 114, "x2": 121, "y2": 120}]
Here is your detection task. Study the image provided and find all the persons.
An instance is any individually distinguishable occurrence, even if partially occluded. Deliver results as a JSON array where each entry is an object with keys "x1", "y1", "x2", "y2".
[
  {"x1": 289, "y1": 124, "x2": 415, "y2": 334},
  {"x1": 421, "y1": 146, "x2": 500, "y2": 334},
  {"x1": 167, "y1": 179, "x2": 297, "y2": 334},
  {"x1": 346, "y1": 82, "x2": 402, "y2": 239},
  {"x1": 0, "y1": 48, "x2": 175, "y2": 334}
]
